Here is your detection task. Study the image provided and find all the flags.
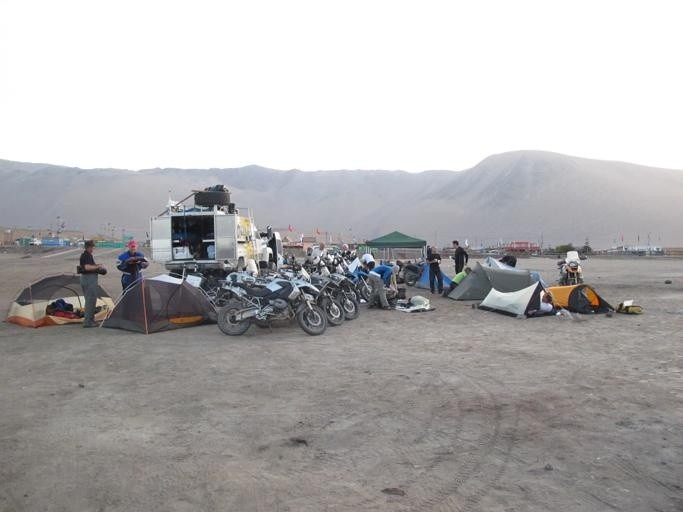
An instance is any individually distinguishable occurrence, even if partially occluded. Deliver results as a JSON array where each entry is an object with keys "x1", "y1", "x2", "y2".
[
  {"x1": 317, "y1": 229, "x2": 320, "y2": 235},
  {"x1": 289, "y1": 224, "x2": 292, "y2": 232}
]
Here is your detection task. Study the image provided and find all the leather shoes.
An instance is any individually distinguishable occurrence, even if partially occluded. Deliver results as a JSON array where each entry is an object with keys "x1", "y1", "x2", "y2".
[{"x1": 83, "y1": 322, "x2": 99, "y2": 328}]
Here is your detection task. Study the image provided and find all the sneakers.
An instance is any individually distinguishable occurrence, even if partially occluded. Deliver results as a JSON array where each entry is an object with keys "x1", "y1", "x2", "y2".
[
  {"x1": 384, "y1": 306, "x2": 392, "y2": 310},
  {"x1": 367, "y1": 305, "x2": 373, "y2": 308}
]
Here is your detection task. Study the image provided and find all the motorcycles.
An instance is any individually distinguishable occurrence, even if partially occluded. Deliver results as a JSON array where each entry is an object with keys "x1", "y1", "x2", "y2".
[
  {"x1": 403, "y1": 260, "x2": 427, "y2": 286},
  {"x1": 216, "y1": 241, "x2": 373, "y2": 336},
  {"x1": 557, "y1": 250, "x2": 587, "y2": 286}
]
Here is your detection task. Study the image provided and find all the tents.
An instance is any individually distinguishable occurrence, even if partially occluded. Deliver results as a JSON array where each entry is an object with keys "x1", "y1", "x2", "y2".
[
  {"x1": 102, "y1": 274, "x2": 218, "y2": 334},
  {"x1": 5, "y1": 274, "x2": 114, "y2": 328},
  {"x1": 414, "y1": 256, "x2": 615, "y2": 319}
]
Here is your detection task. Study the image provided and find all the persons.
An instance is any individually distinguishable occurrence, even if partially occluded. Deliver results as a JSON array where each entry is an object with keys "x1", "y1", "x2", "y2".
[
  {"x1": 311, "y1": 242, "x2": 328, "y2": 258},
  {"x1": 362, "y1": 254, "x2": 375, "y2": 270},
  {"x1": 277, "y1": 253, "x2": 297, "y2": 272},
  {"x1": 451, "y1": 241, "x2": 468, "y2": 275},
  {"x1": 79, "y1": 241, "x2": 107, "y2": 328},
  {"x1": 367, "y1": 265, "x2": 400, "y2": 310},
  {"x1": 426, "y1": 247, "x2": 443, "y2": 294},
  {"x1": 116, "y1": 240, "x2": 149, "y2": 295}
]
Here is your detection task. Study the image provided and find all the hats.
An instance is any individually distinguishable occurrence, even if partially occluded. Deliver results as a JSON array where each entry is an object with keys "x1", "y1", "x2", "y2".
[
  {"x1": 128, "y1": 240, "x2": 138, "y2": 248},
  {"x1": 85, "y1": 241, "x2": 95, "y2": 249}
]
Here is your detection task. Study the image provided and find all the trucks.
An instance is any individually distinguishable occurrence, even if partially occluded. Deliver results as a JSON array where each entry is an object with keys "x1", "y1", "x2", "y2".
[{"x1": 150, "y1": 184, "x2": 273, "y2": 280}]
